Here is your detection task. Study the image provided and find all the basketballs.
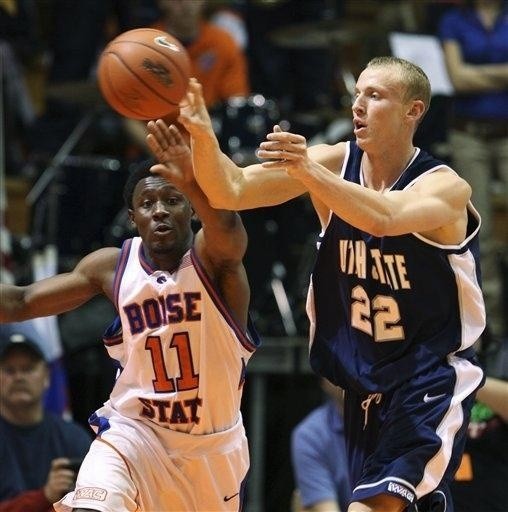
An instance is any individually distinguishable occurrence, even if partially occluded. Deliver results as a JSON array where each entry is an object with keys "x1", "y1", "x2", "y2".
[{"x1": 98, "y1": 28, "x2": 192, "y2": 122}]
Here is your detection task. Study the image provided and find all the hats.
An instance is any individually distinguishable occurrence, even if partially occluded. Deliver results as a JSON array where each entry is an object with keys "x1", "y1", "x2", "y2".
[{"x1": 0, "y1": 332, "x2": 45, "y2": 361}]
